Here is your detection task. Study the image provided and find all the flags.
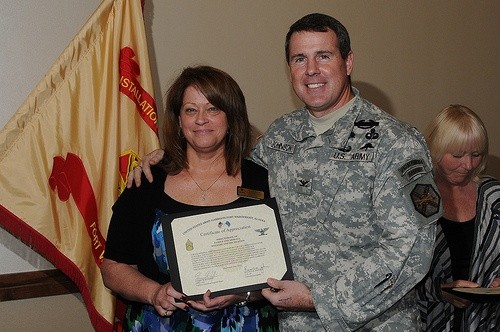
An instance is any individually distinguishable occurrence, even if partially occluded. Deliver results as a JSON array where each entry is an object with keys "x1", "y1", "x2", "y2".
[{"x1": 0, "y1": 0, "x2": 161, "y2": 332}]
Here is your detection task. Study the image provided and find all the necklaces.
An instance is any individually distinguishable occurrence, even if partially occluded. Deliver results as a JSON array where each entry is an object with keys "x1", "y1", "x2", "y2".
[{"x1": 186, "y1": 169, "x2": 226, "y2": 201}]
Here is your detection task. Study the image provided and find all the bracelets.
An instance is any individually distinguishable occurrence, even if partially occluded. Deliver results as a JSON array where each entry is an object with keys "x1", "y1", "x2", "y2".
[{"x1": 235, "y1": 292, "x2": 251, "y2": 306}]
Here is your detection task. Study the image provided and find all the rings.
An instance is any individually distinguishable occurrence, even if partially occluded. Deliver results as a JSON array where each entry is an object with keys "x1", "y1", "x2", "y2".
[
  {"x1": 165, "y1": 311, "x2": 167, "y2": 317},
  {"x1": 166, "y1": 304, "x2": 172, "y2": 310},
  {"x1": 139, "y1": 166, "x2": 142, "y2": 168}
]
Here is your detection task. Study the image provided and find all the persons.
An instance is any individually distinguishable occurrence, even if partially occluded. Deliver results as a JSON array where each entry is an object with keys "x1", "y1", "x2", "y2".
[
  {"x1": 416, "y1": 105, "x2": 500, "y2": 332},
  {"x1": 127, "y1": 13, "x2": 443, "y2": 332},
  {"x1": 101, "y1": 66, "x2": 279, "y2": 332}
]
